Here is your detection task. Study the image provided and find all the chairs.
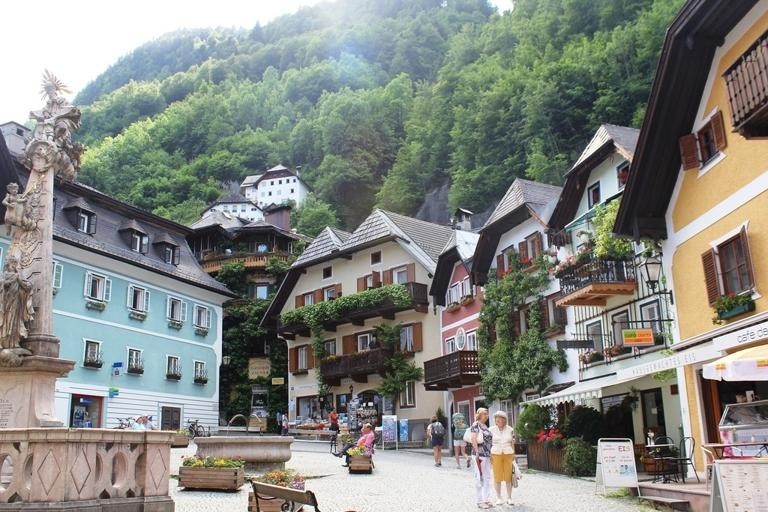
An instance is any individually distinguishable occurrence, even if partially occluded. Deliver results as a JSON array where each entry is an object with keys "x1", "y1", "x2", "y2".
[
  {"x1": 645, "y1": 437, "x2": 699, "y2": 484},
  {"x1": 699, "y1": 442, "x2": 744, "y2": 492}
]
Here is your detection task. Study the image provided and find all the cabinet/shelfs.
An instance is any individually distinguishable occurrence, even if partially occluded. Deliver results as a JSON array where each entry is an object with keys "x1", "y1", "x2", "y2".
[{"x1": 718, "y1": 399, "x2": 768, "y2": 441}]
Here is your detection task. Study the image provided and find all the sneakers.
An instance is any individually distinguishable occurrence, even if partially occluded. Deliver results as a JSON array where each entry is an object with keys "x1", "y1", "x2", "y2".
[
  {"x1": 477, "y1": 498, "x2": 514, "y2": 509},
  {"x1": 434, "y1": 463, "x2": 441, "y2": 467},
  {"x1": 456, "y1": 456, "x2": 471, "y2": 469}
]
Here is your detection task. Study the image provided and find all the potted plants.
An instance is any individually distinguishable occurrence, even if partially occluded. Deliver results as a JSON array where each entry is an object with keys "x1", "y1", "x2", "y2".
[{"x1": 347, "y1": 444, "x2": 374, "y2": 458}]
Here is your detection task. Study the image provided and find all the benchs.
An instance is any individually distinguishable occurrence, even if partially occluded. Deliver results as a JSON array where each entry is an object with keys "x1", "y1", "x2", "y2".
[
  {"x1": 247, "y1": 478, "x2": 356, "y2": 512},
  {"x1": 371, "y1": 439, "x2": 378, "y2": 468}
]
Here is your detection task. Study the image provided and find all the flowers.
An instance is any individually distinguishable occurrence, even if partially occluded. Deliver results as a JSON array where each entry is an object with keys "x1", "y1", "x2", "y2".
[
  {"x1": 260, "y1": 469, "x2": 307, "y2": 491},
  {"x1": 495, "y1": 243, "x2": 587, "y2": 279},
  {"x1": 537, "y1": 429, "x2": 562, "y2": 442},
  {"x1": 577, "y1": 343, "x2": 621, "y2": 366},
  {"x1": 177, "y1": 455, "x2": 245, "y2": 468},
  {"x1": 713, "y1": 291, "x2": 753, "y2": 326}
]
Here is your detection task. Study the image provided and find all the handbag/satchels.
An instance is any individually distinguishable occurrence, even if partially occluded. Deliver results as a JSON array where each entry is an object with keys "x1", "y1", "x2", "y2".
[
  {"x1": 511, "y1": 472, "x2": 518, "y2": 488},
  {"x1": 463, "y1": 428, "x2": 483, "y2": 444}
]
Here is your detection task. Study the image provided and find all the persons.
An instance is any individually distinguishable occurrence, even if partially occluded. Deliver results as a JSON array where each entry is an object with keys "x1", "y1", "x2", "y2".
[
  {"x1": 330, "y1": 407, "x2": 340, "y2": 440},
  {"x1": 487, "y1": 410, "x2": 515, "y2": 506},
  {"x1": 280, "y1": 411, "x2": 288, "y2": 436},
  {"x1": 425, "y1": 415, "x2": 445, "y2": 467},
  {"x1": 332, "y1": 422, "x2": 375, "y2": 467},
  {"x1": 131, "y1": 416, "x2": 146, "y2": 430},
  {"x1": 451, "y1": 412, "x2": 470, "y2": 469},
  {"x1": 0, "y1": 86, "x2": 87, "y2": 349},
  {"x1": 469, "y1": 407, "x2": 496, "y2": 510},
  {"x1": 141, "y1": 414, "x2": 157, "y2": 430}
]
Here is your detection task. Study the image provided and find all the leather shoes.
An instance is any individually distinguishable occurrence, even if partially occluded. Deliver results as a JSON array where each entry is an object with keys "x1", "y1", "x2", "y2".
[
  {"x1": 331, "y1": 451, "x2": 343, "y2": 458},
  {"x1": 341, "y1": 464, "x2": 348, "y2": 468}
]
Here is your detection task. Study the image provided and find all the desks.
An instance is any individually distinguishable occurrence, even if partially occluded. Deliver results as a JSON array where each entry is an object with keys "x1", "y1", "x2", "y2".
[
  {"x1": 288, "y1": 429, "x2": 336, "y2": 440},
  {"x1": 702, "y1": 442, "x2": 768, "y2": 458}
]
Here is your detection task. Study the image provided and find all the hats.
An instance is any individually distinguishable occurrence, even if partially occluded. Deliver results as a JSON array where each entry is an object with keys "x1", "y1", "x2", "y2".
[{"x1": 493, "y1": 411, "x2": 507, "y2": 418}]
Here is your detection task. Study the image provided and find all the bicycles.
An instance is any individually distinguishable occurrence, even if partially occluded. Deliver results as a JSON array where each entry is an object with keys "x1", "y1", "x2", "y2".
[{"x1": 184, "y1": 417, "x2": 205, "y2": 440}]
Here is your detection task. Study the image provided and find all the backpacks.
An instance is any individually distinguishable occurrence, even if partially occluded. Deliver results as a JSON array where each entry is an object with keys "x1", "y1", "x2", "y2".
[{"x1": 431, "y1": 422, "x2": 446, "y2": 439}]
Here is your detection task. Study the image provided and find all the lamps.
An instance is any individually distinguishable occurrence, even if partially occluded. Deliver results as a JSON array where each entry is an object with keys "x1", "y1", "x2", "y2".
[
  {"x1": 638, "y1": 252, "x2": 674, "y2": 304},
  {"x1": 222, "y1": 355, "x2": 231, "y2": 366}
]
